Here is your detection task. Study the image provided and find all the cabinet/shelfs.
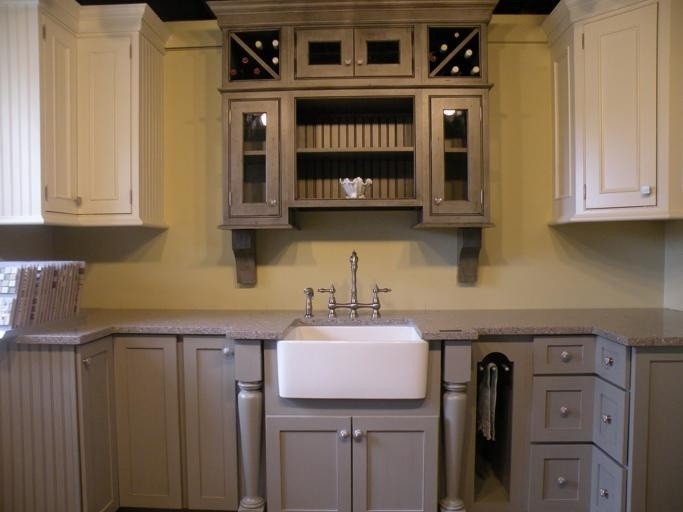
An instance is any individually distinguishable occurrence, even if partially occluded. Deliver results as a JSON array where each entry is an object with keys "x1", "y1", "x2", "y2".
[
  {"x1": 542, "y1": 1, "x2": 683, "y2": 226},
  {"x1": 0, "y1": 335, "x2": 120, "y2": 512},
  {"x1": 263, "y1": 415, "x2": 440, "y2": 512},
  {"x1": 205, "y1": 1, "x2": 500, "y2": 286},
  {"x1": 113, "y1": 333, "x2": 240, "y2": 512},
  {"x1": 0, "y1": 2, "x2": 166, "y2": 229},
  {"x1": 527, "y1": 336, "x2": 683, "y2": 512}
]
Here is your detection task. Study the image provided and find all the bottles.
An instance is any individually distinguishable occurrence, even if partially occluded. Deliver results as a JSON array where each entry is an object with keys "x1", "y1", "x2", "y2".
[
  {"x1": 430, "y1": 32, "x2": 480, "y2": 77},
  {"x1": 230, "y1": 40, "x2": 279, "y2": 80}
]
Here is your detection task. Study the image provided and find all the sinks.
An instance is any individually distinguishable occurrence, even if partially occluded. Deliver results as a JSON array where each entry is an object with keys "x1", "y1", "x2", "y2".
[{"x1": 277, "y1": 325, "x2": 429, "y2": 400}]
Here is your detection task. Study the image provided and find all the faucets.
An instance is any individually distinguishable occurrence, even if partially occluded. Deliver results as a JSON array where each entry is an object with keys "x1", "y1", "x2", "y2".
[
  {"x1": 304, "y1": 288, "x2": 313, "y2": 319},
  {"x1": 349, "y1": 251, "x2": 359, "y2": 318}
]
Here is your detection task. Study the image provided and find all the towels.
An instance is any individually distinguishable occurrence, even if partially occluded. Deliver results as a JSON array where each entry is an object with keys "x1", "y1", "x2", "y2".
[{"x1": 477, "y1": 363, "x2": 498, "y2": 441}]
